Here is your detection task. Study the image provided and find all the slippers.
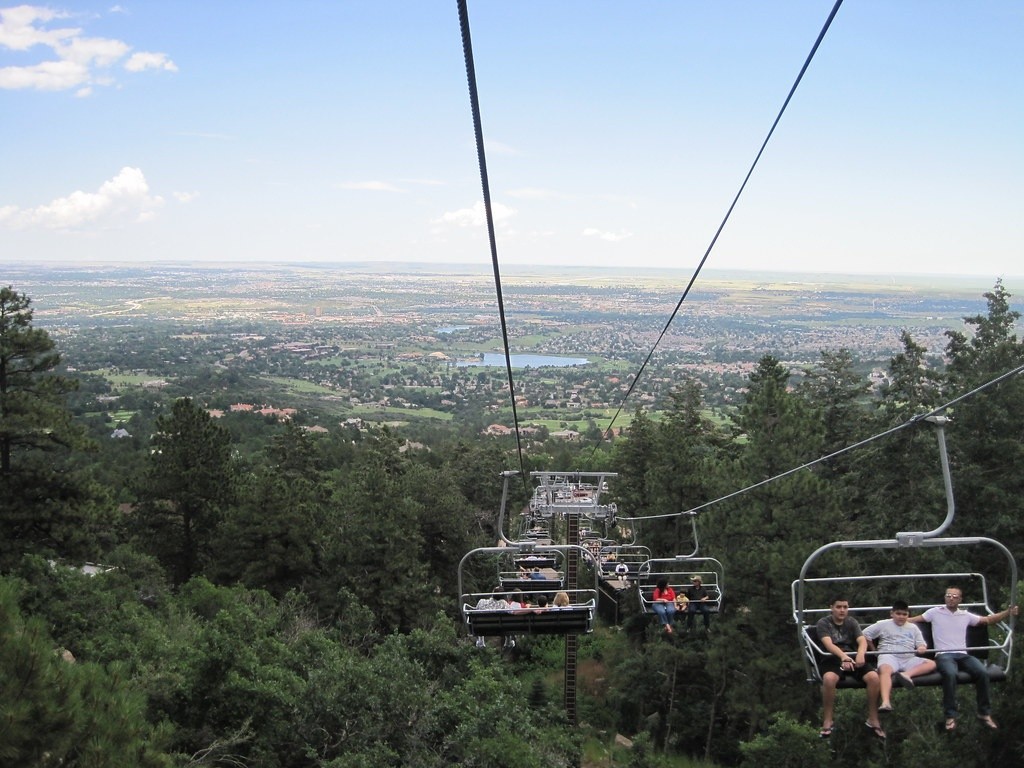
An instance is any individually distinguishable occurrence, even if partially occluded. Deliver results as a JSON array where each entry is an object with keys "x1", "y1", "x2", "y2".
[
  {"x1": 899, "y1": 672, "x2": 914, "y2": 690},
  {"x1": 979, "y1": 717, "x2": 996, "y2": 729},
  {"x1": 865, "y1": 722, "x2": 886, "y2": 739},
  {"x1": 819, "y1": 727, "x2": 834, "y2": 738},
  {"x1": 877, "y1": 707, "x2": 893, "y2": 712},
  {"x1": 946, "y1": 719, "x2": 955, "y2": 730}
]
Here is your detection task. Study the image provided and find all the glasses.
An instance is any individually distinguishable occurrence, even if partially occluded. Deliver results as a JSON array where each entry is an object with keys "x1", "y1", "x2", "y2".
[{"x1": 944, "y1": 593, "x2": 961, "y2": 600}]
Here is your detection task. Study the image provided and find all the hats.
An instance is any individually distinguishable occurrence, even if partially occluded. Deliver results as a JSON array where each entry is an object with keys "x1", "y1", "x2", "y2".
[{"x1": 690, "y1": 576, "x2": 703, "y2": 583}]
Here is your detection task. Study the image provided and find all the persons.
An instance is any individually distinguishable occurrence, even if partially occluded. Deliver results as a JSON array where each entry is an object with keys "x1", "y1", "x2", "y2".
[
  {"x1": 474, "y1": 518, "x2": 628, "y2": 647},
  {"x1": 814, "y1": 596, "x2": 887, "y2": 740},
  {"x1": 907, "y1": 585, "x2": 1019, "y2": 732},
  {"x1": 652, "y1": 575, "x2": 712, "y2": 634},
  {"x1": 862, "y1": 598, "x2": 936, "y2": 713}
]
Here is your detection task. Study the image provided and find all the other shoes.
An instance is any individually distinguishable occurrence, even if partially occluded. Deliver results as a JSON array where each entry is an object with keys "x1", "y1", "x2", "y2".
[
  {"x1": 686, "y1": 627, "x2": 691, "y2": 633},
  {"x1": 476, "y1": 640, "x2": 485, "y2": 648},
  {"x1": 504, "y1": 640, "x2": 515, "y2": 648},
  {"x1": 706, "y1": 629, "x2": 711, "y2": 633},
  {"x1": 665, "y1": 625, "x2": 672, "y2": 634}
]
